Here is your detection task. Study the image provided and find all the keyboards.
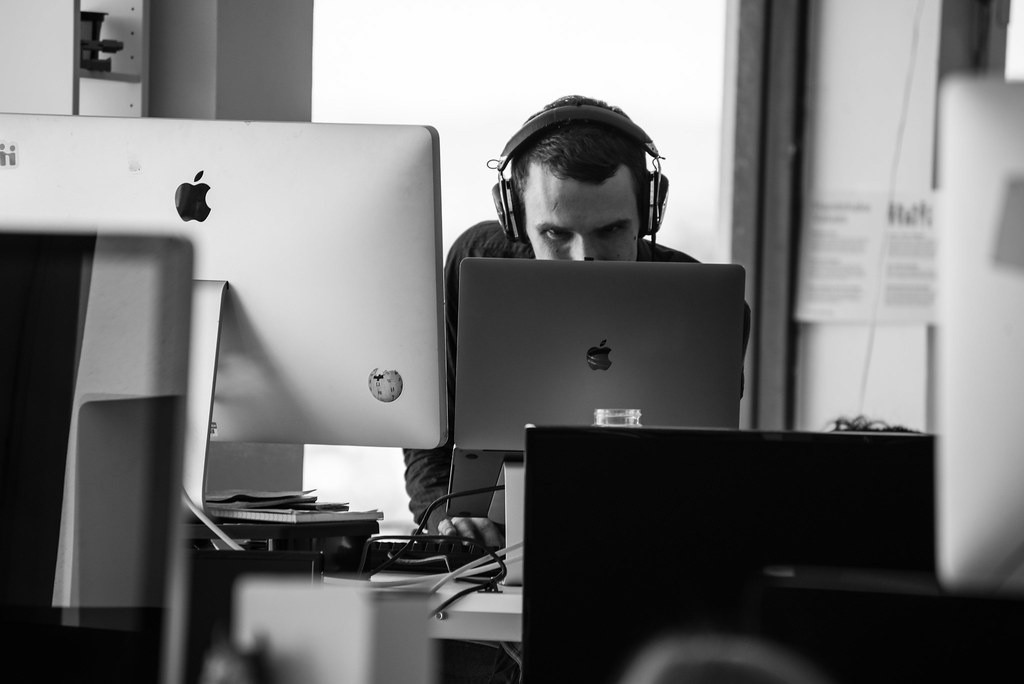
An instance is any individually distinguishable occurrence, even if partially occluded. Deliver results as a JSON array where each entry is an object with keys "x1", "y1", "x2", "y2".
[{"x1": 337, "y1": 536, "x2": 506, "y2": 574}]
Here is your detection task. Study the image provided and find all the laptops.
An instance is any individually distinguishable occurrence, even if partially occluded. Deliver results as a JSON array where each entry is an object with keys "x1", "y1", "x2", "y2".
[
  {"x1": 447, "y1": 257, "x2": 746, "y2": 518},
  {"x1": 519, "y1": 418, "x2": 935, "y2": 684}
]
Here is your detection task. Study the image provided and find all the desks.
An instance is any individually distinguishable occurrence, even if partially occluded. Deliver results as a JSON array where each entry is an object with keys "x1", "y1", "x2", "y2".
[{"x1": 322, "y1": 562, "x2": 522, "y2": 644}]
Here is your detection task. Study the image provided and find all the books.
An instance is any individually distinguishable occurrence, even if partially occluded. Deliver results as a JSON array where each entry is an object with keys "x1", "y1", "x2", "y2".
[{"x1": 205, "y1": 490, "x2": 385, "y2": 525}]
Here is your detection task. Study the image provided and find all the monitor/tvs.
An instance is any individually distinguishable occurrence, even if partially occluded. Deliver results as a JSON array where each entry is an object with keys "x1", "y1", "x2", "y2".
[
  {"x1": 0, "y1": 223, "x2": 194, "y2": 684},
  {"x1": 0, "y1": 113, "x2": 447, "y2": 536},
  {"x1": 937, "y1": 72, "x2": 1024, "y2": 597}
]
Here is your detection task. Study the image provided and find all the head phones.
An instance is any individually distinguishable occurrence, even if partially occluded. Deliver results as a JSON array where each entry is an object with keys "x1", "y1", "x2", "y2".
[{"x1": 491, "y1": 104, "x2": 669, "y2": 243}]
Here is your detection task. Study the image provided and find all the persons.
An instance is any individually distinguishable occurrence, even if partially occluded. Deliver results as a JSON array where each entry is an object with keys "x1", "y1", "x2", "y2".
[{"x1": 399, "y1": 94, "x2": 753, "y2": 546}]
